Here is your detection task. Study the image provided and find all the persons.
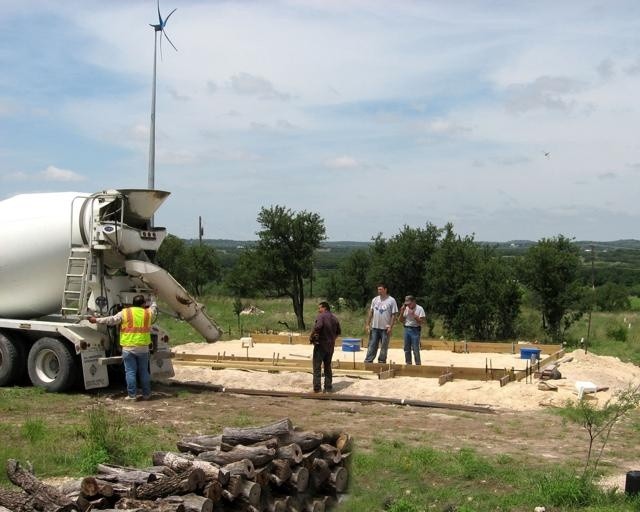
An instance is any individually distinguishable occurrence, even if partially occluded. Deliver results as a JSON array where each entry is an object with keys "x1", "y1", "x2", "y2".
[
  {"x1": 87, "y1": 294, "x2": 158, "y2": 402},
  {"x1": 398, "y1": 295, "x2": 426, "y2": 365},
  {"x1": 307, "y1": 302, "x2": 341, "y2": 393},
  {"x1": 364, "y1": 284, "x2": 398, "y2": 363}
]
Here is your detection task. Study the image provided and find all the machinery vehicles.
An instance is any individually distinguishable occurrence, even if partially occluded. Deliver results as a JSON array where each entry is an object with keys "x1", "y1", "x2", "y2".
[{"x1": 0, "y1": 188, "x2": 223, "y2": 395}]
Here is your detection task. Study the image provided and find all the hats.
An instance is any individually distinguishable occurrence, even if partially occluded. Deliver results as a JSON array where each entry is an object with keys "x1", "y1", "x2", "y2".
[{"x1": 405, "y1": 295, "x2": 413, "y2": 305}]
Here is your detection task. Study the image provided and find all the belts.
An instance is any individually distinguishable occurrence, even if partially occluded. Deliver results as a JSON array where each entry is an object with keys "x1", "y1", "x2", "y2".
[{"x1": 405, "y1": 326, "x2": 420, "y2": 328}]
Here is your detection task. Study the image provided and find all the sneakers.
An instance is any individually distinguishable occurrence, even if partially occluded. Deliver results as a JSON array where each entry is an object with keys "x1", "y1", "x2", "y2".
[{"x1": 124, "y1": 393, "x2": 152, "y2": 401}]
